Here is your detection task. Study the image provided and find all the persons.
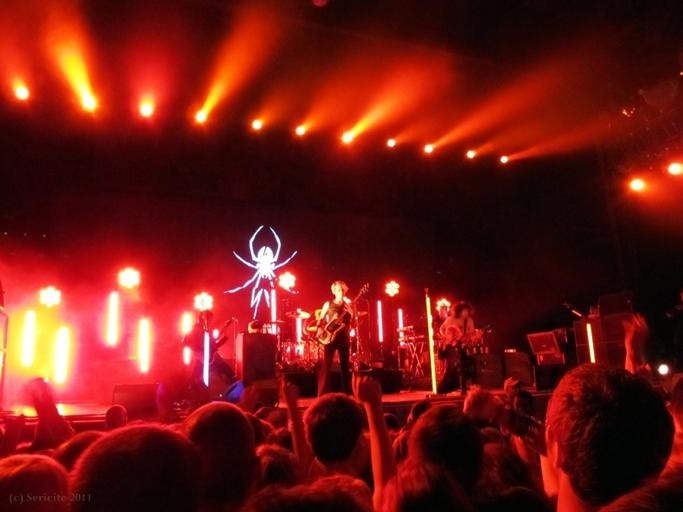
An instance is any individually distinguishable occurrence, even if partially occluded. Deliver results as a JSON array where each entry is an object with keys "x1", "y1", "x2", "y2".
[
  {"x1": 440, "y1": 302, "x2": 480, "y2": 394},
  {"x1": 317, "y1": 280, "x2": 353, "y2": 394},
  {"x1": 166, "y1": 310, "x2": 238, "y2": 392},
  {"x1": 559, "y1": 310, "x2": 577, "y2": 367},
  {"x1": 302, "y1": 319, "x2": 314, "y2": 368},
  {"x1": 307, "y1": 310, "x2": 322, "y2": 330}
]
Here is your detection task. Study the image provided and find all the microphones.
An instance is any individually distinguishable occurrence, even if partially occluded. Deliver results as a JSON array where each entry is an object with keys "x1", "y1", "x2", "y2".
[{"x1": 561, "y1": 302, "x2": 583, "y2": 318}]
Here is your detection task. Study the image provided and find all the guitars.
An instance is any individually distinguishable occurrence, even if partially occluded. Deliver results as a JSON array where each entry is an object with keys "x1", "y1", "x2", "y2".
[
  {"x1": 200, "y1": 317, "x2": 237, "y2": 366},
  {"x1": 316, "y1": 282, "x2": 370, "y2": 346},
  {"x1": 455, "y1": 324, "x2": 496, "y2": 346}
]
